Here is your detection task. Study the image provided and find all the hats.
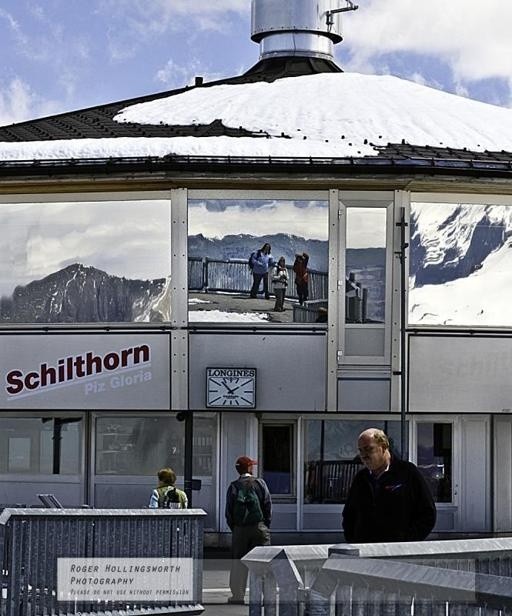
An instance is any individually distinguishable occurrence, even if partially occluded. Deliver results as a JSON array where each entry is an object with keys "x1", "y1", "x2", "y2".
[
  {"x1": 234, "y1": 456, "x2": 257, "y2": 469},
  {"x1": 294, "y1": 253, "x2": 303, "y2": 257}
]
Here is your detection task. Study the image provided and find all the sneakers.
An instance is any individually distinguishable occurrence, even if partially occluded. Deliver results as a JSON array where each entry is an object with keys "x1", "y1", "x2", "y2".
[
  {"x1": 249, "y1": 293, "x2": 257, "y2": 299},
  {"x1": 228, "y1": 595, "x2": 245, "y2": 604},
  {"x1": 264, "y1": 295, "x2": 270, "y2": 300}
]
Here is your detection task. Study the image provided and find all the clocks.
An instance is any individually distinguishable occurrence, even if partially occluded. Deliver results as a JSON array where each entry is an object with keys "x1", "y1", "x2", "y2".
[{"x1": 206, "y1": 367, "x2": 256, "y2": 409}]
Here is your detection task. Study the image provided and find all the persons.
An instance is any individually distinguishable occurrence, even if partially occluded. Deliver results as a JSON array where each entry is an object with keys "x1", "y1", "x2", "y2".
[
  {"x1": 149, "y1": 469, "x2": 189, "y2": 514},
  {"x1": 315, "y1": 307, "x2": 328, "y2": 323},
  {"x1": 224, "y1": 456, "x2": 273, "y2": 606},
  {"x1": 340, "y1": 428, "x2": 438, "y2": 543},
  {"x1": 249, "y1": 242, "x2": 270, "y2": 300},
  {"x1": 293, "y1": 252, "x2": 309, "y2": 306},
  {"x1": 270, "y1": 256, "x2": 290, "y2": 311}
]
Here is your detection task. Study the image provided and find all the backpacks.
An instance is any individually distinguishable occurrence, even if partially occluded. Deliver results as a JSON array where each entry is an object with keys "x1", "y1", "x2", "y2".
[
  {"x1": 248, "y1": 249, "x2": 262, "y2": 271},
  {"x1": 230, "y1": 477, "x2": 265, "y2": 526}
]
